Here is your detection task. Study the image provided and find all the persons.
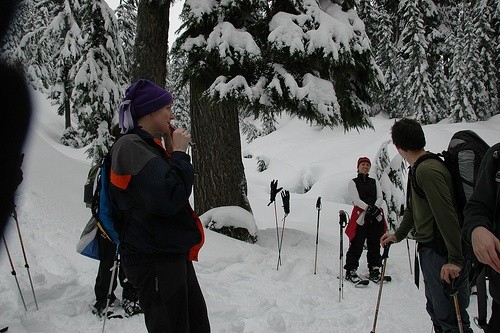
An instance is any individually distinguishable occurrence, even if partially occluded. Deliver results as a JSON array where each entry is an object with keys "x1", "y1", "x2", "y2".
[
  {"x1": 343, "y1": 157, "x2": 389, "y2": 282},
  {"x1": 0, "y1": 0, "x2": 34, "y2": 268},
  {"x1": 461, "y1": 141, "x2": 500, "y2": 333},
  {"x1": 381, "y1": 119, "x2": 473, "y2": 333},
  {"x1": 75, "y1": 78, "x2": 211, "y2": 333}
]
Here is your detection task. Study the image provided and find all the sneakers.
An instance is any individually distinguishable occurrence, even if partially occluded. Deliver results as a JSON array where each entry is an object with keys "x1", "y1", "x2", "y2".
[
  {"x1": 368, "y1": 267, "x2": 381, "y2": 282},
  {"x1": 346, "y1": 270, "x2": 361, "y2": 284}
]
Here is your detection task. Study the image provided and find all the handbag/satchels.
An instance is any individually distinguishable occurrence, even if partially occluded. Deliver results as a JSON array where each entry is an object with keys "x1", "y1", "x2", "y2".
[{"x1": 76, "y1": 217, "x2": 101, "y2": 260}]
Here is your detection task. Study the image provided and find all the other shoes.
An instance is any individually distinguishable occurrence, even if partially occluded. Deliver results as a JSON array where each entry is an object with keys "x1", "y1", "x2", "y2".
[
  {"x1": 94, "y1": 302, "x2": 122, "y2": 319},
  {"x1": 122, "y1": 298, "x2": 140, "y2": 314}
]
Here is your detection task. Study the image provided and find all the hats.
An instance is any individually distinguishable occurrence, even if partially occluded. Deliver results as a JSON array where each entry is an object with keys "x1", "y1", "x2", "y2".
[
  {"x1": 118, "y1": 79, "x2": 172, "y2": 135},
  {"x1": 357, "y1": 157, "x2": 371, "y2": 167}
]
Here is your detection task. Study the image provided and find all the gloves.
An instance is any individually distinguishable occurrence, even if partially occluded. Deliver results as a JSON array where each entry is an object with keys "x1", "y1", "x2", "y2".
[
  {"x1": 281, "y1": 190, "x2": 290, "y2": 213},
  {"x1": 266, "y1": 179, "x2": 283, "y2": 206}
]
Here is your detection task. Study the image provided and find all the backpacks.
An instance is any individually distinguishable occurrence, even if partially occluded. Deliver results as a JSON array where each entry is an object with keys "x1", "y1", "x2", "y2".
[
  {"x1": 411, "y1": 129, "x2": 491, "y2": 228},
  {"x1": 84, "y1": 161, "x2": 103, "y2": 209}
]
